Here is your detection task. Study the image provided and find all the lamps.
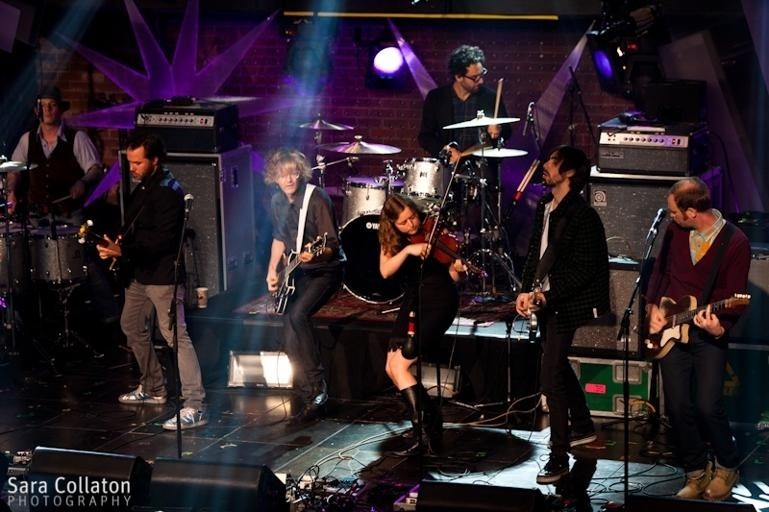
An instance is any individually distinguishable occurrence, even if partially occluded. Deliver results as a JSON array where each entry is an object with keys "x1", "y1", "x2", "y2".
[
  {"x1": 586, "y1": 19, "x2": 658, "y2": 99},
  {"x1": 226, "y1": 350, "x2": 295, "y2": 389},
  {"x1": 364, "y1": 30, "x2": 426, "y2": 90}
]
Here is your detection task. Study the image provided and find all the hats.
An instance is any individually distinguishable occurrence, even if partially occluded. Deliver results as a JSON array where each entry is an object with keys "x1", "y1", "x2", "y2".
[{"x1": 33, "y1": 83, "x2": 71, "y2": 111}]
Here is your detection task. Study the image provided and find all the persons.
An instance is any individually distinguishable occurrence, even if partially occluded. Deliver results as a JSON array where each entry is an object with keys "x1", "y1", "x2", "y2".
[
  {"x1": 5, "y1": 89, "x2": 104, "y2": 226},
  {"x1": 376, "y1": 192, "x2": 472, "y2": 461},
  {"x1": 94, "y1": 132, "x2": 211, "y2": 431},
  {"x1": 263, "y1": 148, "x2": 345, "y2": 427},
  {"x1": 416, "y1": 47, "x2": 512, "y2": 203},
  {"x1": 515, "y1": 146, "x2": 612, "y2": 485},
  {"x1": 642, "y1": 177, "x2": 751, "y2": 500}
]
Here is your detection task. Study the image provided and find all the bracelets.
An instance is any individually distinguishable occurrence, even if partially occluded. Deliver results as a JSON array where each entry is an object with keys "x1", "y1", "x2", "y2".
[{"x1": 715, "y1": 328, "x2": 723, "y2": 339}]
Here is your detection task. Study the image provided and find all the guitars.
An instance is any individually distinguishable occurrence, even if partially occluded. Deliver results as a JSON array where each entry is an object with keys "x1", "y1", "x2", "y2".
[
  {"x1": 75, "y1": 221, "x2": 128, "y2": 276},
  {"x1": 272, "y1": 233, "x2": 326, "y2": 315},
  {"x1": 527, "y1": 287, "x2": 542, "y2": 342},
  {"x1": 641, "y1": 295, "x2": 749, "y2": 360}
]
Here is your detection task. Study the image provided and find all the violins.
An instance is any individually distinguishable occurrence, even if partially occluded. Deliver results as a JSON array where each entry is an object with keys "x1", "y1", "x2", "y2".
[{"x1": 411, "y1": 216, "x2": 488, "y2": 277}]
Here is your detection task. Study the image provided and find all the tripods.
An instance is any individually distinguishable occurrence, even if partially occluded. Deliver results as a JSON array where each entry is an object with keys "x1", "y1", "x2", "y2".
[{"x1": 388, "y1": 285, "x2": 438, "y2": 482}]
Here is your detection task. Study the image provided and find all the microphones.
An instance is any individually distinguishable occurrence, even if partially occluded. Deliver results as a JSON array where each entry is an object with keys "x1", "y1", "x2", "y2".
[
  {"x1": 646, "y1": 208, "x2": 668, "y2": 241},
  {"x1": 183, "y1": 193, "x2": 195, "y2": 223}
]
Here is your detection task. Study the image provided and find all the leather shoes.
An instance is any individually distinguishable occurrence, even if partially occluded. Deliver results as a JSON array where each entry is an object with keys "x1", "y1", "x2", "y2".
[
  {"x1": 703, "y1": 460, "x2": 739, "y2": 501},
  {"x1": 676, "y1": 462, "x2": 711, "y2": 499}
]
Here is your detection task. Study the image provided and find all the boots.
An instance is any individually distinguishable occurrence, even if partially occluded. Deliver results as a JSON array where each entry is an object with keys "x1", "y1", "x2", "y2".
[{"x1": 397, "y1": 383, "x2": 448, "y2": 463}]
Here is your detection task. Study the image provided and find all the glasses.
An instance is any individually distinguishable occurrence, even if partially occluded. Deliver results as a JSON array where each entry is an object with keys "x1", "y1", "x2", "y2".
[{"x1": 461, "y1": 68, "x2": 487, "y2": 83}]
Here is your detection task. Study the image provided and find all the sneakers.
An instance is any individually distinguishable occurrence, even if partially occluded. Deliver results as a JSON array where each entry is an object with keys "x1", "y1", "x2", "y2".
[
  {"x1": 548, "y1": 421, "x2": 596, "y2": 449},
  {"x1": 537, "y1": 458, "x2": 569, "y2": 485},
  {"x1": 162, "y1": 406, "x2": 208, "y2": 430},
  {"x1": 312, "y1": 380, "x2": 328, "y2": 406},
  {"x1": 118, "y1": 384, "x2": 167, "y2": 404},
  {"x1": 303, "y1": 402, "x2": 322, "y2": 420}
]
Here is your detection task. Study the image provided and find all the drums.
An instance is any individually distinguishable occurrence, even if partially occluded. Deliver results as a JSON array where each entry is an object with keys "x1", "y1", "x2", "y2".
[
  {"x1": 0, "y1": 221, "x2": 25, "y2": 286},
  {"x1": 338, "y1": 213, "x2": 417, "y2": 305},
  {"x1": 403, "y1": 157, "x2": 448, "y2": 203},
  {"x1": 342, "y1": 177, "x2": 388, "y2": 224},
  {"x1": 30, "y1": 226, "x2": 83, "y2": 285}
]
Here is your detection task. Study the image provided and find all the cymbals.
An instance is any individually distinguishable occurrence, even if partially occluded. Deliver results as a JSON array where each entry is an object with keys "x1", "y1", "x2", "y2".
[
  {"x1": 472, "y1": 149, "x2": 528, "y2": 158},
  {"x1": 299, "y1": 118, "x2": 353, "y2": 131},
  {"x1": 443, "y1": 111, "x2": 520, "y2": 129},
  {"x1": 0, "y1": 161, "x2": 36, "y2": 171},
  {"x1": 316, "y1": 136, "x2": 402, "y2": 154}
]
